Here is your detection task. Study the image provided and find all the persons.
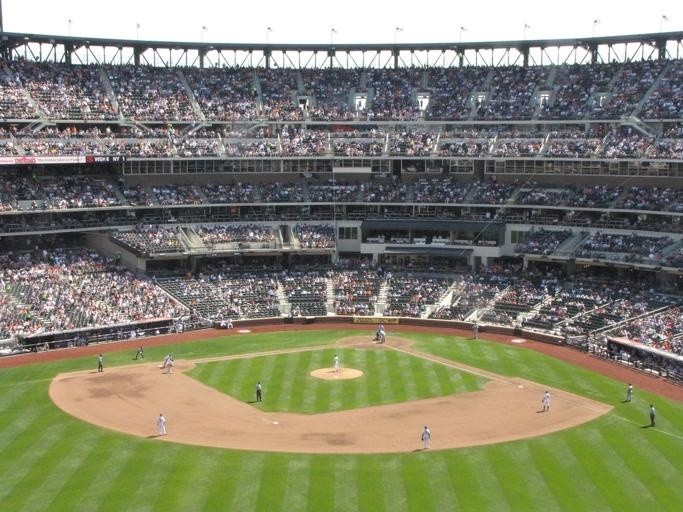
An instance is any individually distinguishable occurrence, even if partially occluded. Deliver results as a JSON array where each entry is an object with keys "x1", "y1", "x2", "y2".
[
  {"x1": 255, "y1": 381, "x2": 263, "y2": 401},
  {"x1": 0, "y1": 56, "x2": 682, "y2": 379},
  {"x1": 156, "y1": 413, "x2": 166, "y2": 434},
  {"x1": 421, "y1": 425, "x2": 431, "y2": 449},
  {"x1": 540, "y1": 390, "x2": 550, "y2": 411},
  {"x1": 649, "y1": 403, "x2": 655, "y2": 427},
  {"x1": 625, "y1": 383, "x2": 633, "y2": 402}
]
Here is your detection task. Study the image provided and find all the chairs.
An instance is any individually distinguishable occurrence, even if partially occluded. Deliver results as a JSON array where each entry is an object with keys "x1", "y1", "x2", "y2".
[
  {"x1": 0, "y1": 173, "x2": 682, "y2": 384},
  {"x1": 0, "y1": 54, "x2": 679, "y2": 178}
]
